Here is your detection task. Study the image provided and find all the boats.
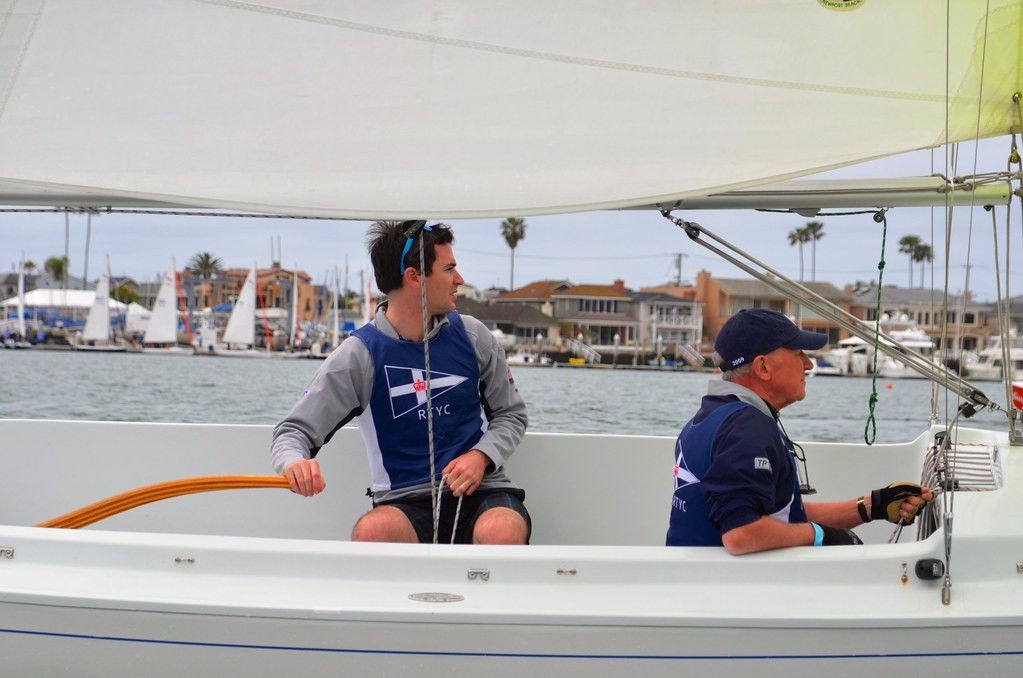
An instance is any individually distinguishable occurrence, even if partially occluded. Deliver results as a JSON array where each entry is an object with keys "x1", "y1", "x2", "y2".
[
  {"x1": 803, "y1": 356, "x2": 844, "y2": 376},
  {"x1": 831, "y1": 312, "x2": 957, "y2": 381},
  {"x1": 962, "y1": 329, "x2": 1023, "y2": 382},
  {"x1": 506, "y1": 351, "x2": 554, "y2": 368}
]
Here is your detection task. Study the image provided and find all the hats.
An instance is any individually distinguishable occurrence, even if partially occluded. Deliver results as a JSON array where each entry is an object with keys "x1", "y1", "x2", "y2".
[{"x1": 714, "y1": 308, "x2": 830, "y2": 372}]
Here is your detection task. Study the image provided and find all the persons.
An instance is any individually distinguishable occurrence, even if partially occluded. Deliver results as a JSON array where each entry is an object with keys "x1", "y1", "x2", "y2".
[
  {"x1": 270, "y1": 220, "x2": 533, "y2": 545},
  {"x1": 666, "y1": 308, "x2": 934, "y2": 555}
]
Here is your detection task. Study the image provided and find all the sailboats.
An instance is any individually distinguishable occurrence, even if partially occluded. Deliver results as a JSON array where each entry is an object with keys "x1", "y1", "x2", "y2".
[{"x1": 1, "y1": 253, "x2": 371, "y2": 360}]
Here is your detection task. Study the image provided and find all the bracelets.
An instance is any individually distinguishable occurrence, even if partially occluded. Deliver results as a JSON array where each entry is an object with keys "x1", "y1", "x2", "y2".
[{"x1": 857, "y1": 495, "x2": 871, "y2": 524}]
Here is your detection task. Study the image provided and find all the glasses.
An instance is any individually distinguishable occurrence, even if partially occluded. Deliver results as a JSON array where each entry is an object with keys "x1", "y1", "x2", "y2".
[{"x1": 401, "y1": 225, "x2": 432, "y2": 276}]
